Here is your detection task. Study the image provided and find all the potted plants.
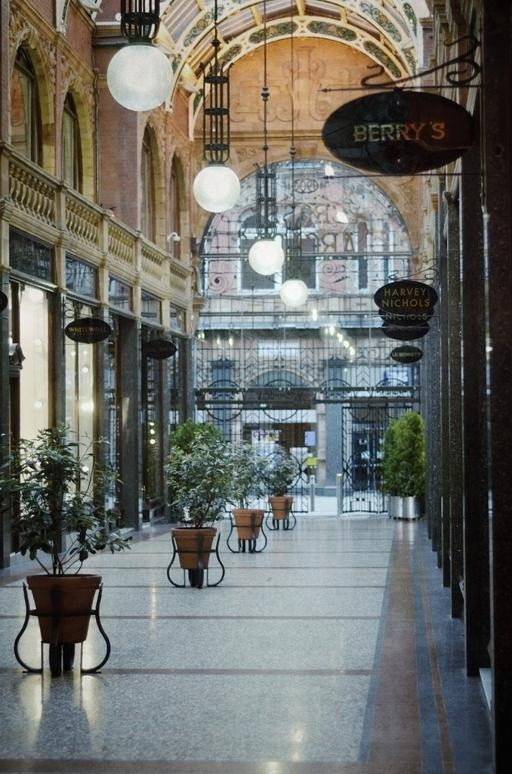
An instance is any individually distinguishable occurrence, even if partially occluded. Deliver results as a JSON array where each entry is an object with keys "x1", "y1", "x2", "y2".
[
  {"x1": 158, "y1": 416, "x2": 299, "y2": 569},
  {"x1": 377, "y1": 407, "x2": 426, "y2": 520},
  {"x1": 0, "y1": 417, "x2": 136, "y2": 645}
]
